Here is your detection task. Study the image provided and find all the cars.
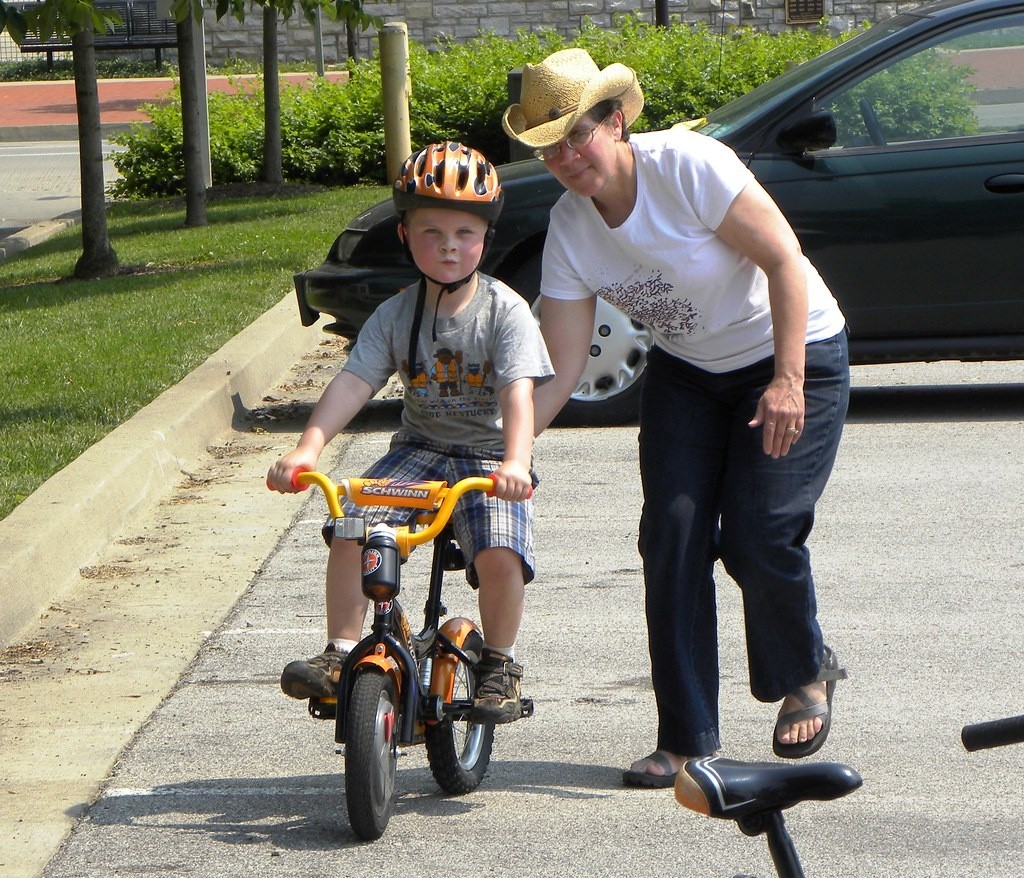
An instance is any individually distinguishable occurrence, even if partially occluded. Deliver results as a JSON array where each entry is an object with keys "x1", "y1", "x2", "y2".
[{"x1": 307, "y1": 1, "x2": 1024, "y2": 422}]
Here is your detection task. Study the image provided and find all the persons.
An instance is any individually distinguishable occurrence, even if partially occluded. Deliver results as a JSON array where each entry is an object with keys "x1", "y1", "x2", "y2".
[
  {"x1": 267, "y1": 138, "x2": 557, "y2": 724},
  {"x1": 527, "y1": 47, "x2": 854, "y2": 789}
]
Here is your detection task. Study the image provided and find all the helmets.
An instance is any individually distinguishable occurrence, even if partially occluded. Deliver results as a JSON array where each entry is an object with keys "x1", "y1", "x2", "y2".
[{"x1": 393, "y1": 141, "x2": 504, "y2": 227}]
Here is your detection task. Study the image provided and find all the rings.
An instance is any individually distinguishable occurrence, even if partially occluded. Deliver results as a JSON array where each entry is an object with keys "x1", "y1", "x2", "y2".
[{"x1": 786, "y1": 427, "x2": 800, "y2": 435}]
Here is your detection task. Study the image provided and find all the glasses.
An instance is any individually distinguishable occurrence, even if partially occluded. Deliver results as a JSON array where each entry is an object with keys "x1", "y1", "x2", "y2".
[{"x1": 533, "y1": 115, "x2": 608, "y2": 161}]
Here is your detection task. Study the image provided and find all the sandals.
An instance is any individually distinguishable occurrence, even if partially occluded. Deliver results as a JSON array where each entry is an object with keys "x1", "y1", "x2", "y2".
[
  {"x1": 622, "y1": 750, "x2": 717, "y2": 788},
  {"x1": 773, "y1": 645, "x2": 848, "y2": 759}
]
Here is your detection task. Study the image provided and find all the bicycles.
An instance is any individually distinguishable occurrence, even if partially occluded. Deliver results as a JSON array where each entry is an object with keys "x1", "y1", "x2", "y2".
[{"x1": 264, "y1": 465, "x2": 536, "y2": 841}]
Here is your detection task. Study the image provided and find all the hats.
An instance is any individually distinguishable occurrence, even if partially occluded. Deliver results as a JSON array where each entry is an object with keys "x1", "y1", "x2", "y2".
[{"x1": 502, "y1": 48, "x2": 644, "y2": 146}]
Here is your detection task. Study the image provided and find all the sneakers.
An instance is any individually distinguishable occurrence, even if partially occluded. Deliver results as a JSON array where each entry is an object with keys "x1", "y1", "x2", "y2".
[
  {"x1": 281, "y1": 644, "x2": 350, "y2": 701},
  {"x1": 471, "y1": 648, "x2": 523, "y2": 723}
]
